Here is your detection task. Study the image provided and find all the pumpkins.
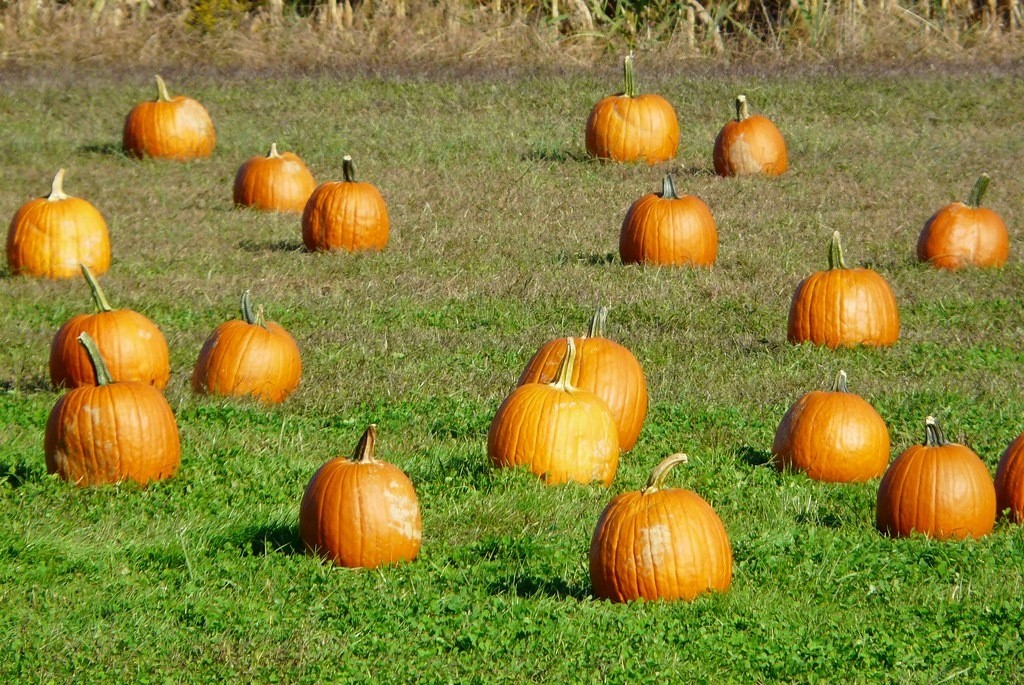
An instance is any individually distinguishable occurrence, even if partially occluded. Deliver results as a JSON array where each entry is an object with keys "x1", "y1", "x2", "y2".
[{"x1": 8, "y1": 53, "x2": 1024, "y2": 607}]
